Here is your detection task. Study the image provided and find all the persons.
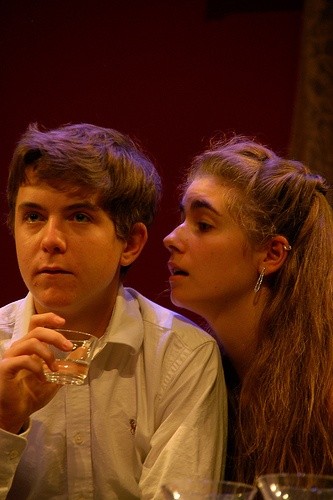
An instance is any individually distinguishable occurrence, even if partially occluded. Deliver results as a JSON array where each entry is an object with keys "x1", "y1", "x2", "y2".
[
  {"x1": 163, "y1": 139, "x2": 333, "y2": 500},
  {"x1": 0, "y1": 123, "x2": 228, "y2": 500}
]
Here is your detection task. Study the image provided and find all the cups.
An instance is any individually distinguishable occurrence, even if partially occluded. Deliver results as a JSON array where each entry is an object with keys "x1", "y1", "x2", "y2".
[
  {"x1": 39, "y1": 329, "x2": 98, "y2": 385},
  {"x1": 257, "y1": 473, "x2": 333, "y2": 500},
  {"x1": 160, "y1": 480, "x2": 256, "y2": 500}
]
